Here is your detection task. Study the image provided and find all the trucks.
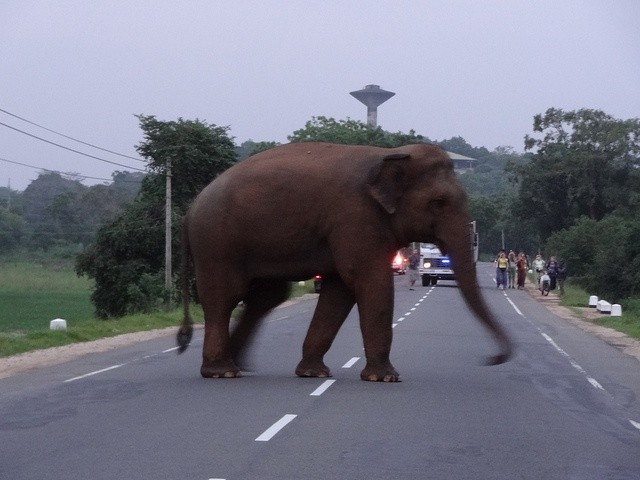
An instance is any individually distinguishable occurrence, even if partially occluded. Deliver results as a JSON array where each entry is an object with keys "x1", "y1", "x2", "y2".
[{"x1": 419, "y1": 229, "x2": 478, "y2": 286}]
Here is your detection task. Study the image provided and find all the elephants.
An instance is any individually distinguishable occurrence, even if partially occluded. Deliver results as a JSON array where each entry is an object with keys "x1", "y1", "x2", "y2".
[{"x1": 174, "y1": 140, "x2": 519, "y2": 383}]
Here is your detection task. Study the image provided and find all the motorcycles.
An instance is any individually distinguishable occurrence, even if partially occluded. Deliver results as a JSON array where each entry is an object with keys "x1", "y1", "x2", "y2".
[{"x1": 536, "y1": 262, "x2": 551, "y2": 296}]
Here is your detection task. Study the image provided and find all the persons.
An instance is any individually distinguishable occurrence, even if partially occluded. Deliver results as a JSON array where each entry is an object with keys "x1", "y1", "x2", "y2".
[
  {"x1": 407, "y1": 248, "x2": 420, "y2": 291},
  {"x1": 547, "y1": 260, "x2": 559, "y2": 290},
  {"x1": 532, "y1": 255, "x2": 546, "y2": 290},
  {"x1": 558, "y1": 260, "x2": 567, "y2": 298},
  {"x1": 495, "y1": 249, "x2": 528, "y2": 290}
]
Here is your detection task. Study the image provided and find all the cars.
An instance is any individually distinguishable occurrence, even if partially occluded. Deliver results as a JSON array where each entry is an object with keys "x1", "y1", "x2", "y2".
[{"x1": 391, "y1": 253, "x2": 406, "y2": 274}]
